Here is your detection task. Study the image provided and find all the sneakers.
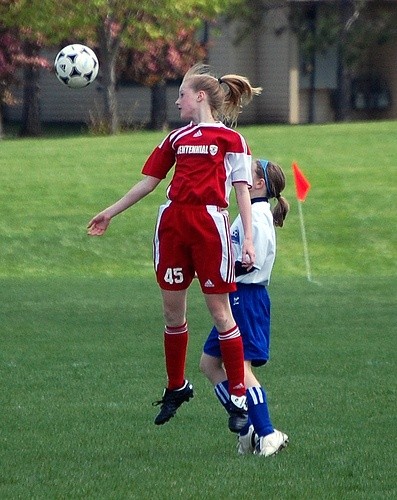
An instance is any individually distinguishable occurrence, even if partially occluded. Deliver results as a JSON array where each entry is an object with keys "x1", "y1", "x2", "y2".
[
  {"x1": 228, "y1": 394, "x2": 248, "y2": 432},
  {"x1": 151, "y1": 379, "x2": 193, "y2": 425},
  {"x1": 236, "y1": 424, "x2": 258, "y2": 455},
  {"x1": 253, "y1": 429, "x2": 289, "y2": 458}
]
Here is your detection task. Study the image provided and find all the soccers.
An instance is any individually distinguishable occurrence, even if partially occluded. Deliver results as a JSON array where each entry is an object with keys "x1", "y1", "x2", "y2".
[{"x1": 54, "y1": 44, "x2": 99, "y2": 88}]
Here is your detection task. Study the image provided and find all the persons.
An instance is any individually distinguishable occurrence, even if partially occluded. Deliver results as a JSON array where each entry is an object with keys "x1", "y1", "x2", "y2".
[
  {"x1": 198, "y1": 158, "x2": 291, "y2": 458},
  {"x1": 87, "y1": 61, "x2": 264, "y2": 433}
]
